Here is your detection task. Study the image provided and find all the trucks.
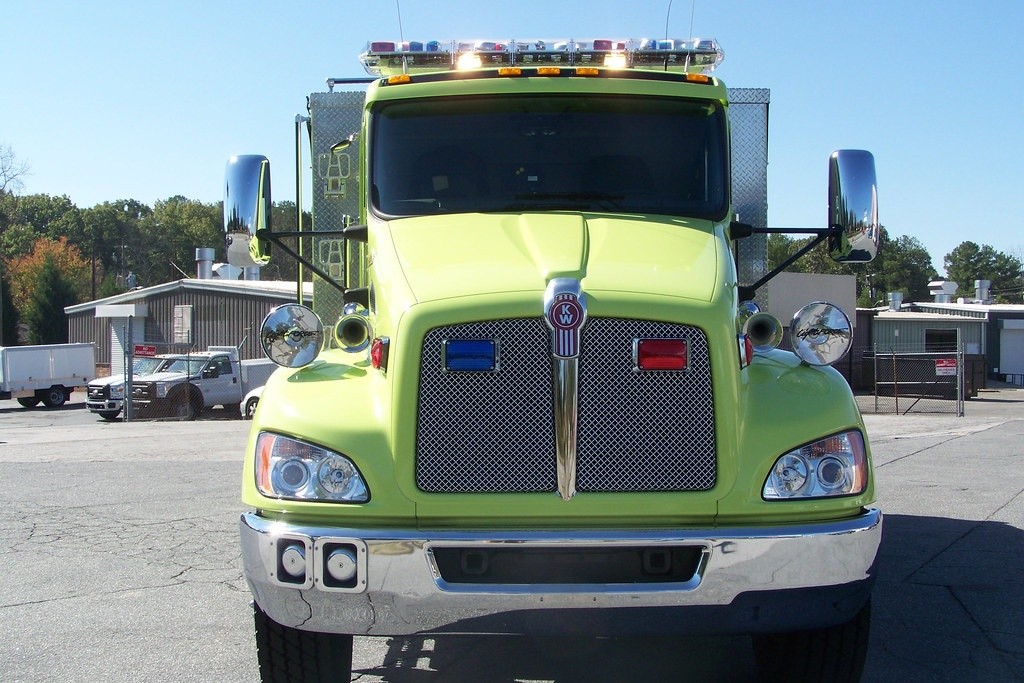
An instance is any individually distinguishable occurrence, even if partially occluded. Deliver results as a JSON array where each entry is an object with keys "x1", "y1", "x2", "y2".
[
  {"x1": 224, "y1": 36, "x2": 882, "y2": 683},
  {"x1": 86, "y1": 354, "x2": 187, "y2": 419},
  {"x1": 130, "y1": 345, "x2": 280, "y2": 422}
]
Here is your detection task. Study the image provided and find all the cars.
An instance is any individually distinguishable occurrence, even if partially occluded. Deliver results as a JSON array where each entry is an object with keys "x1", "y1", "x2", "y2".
[{"x1": 239, "y1": 384, "x2": 265, "y2": 420}]
